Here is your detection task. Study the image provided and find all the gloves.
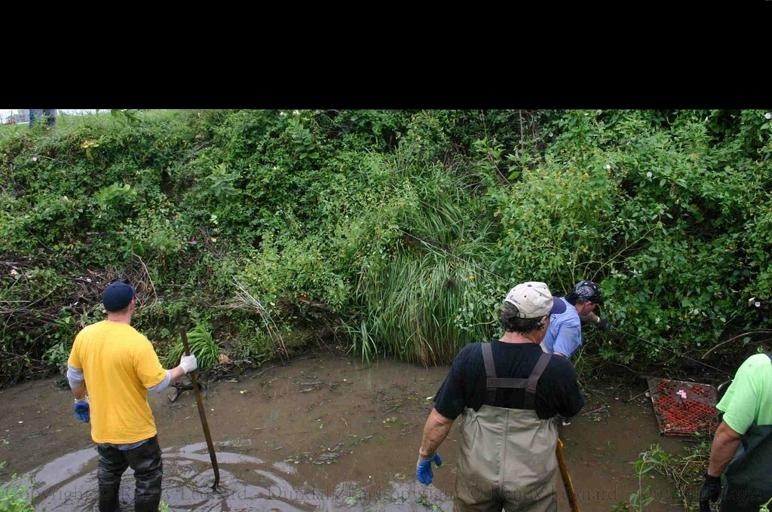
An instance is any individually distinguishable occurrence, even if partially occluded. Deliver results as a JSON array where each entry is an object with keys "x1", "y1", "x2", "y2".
[
  {"x1": 595, "y1": 316, "x2": 609, "y2": 332},
  {"x1": 416, "y1": 454, "x2": 441, "y2": 485},
  {"x1": 700, "y1": 473, "x2": 721, "y2": 512},
  {"x1": 74, "y1": 395, "x2": 90, "y2": 423},
  {"x1": 180, "y1": 352, "x2": 197, "y2": 375}
]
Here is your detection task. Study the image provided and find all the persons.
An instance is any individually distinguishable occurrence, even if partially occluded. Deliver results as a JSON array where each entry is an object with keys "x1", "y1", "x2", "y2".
[
  {"x1": 415, "y1": 280, "x2": 584, "y2": 511},
  {"x1": 698, "y1": 349, "x2": 771, "y2": 511},
  {"x1": 65, "y1": 278, "x2": 199, "y2": 512},
  {"x1": 537, "y1": 278, "x2": 609, "y2": 364}
]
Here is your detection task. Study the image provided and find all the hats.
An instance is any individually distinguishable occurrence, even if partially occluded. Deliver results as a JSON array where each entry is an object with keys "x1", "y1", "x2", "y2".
[
  {"x1": 103, "y1": 282, "x2": 136, "y2": 310},
  {"x1": 501, "y1": 281, "x2": 566, "y2": 319},
  {"x1": 573, "y1": 281, "x2": 602, "y2": 298}
]
